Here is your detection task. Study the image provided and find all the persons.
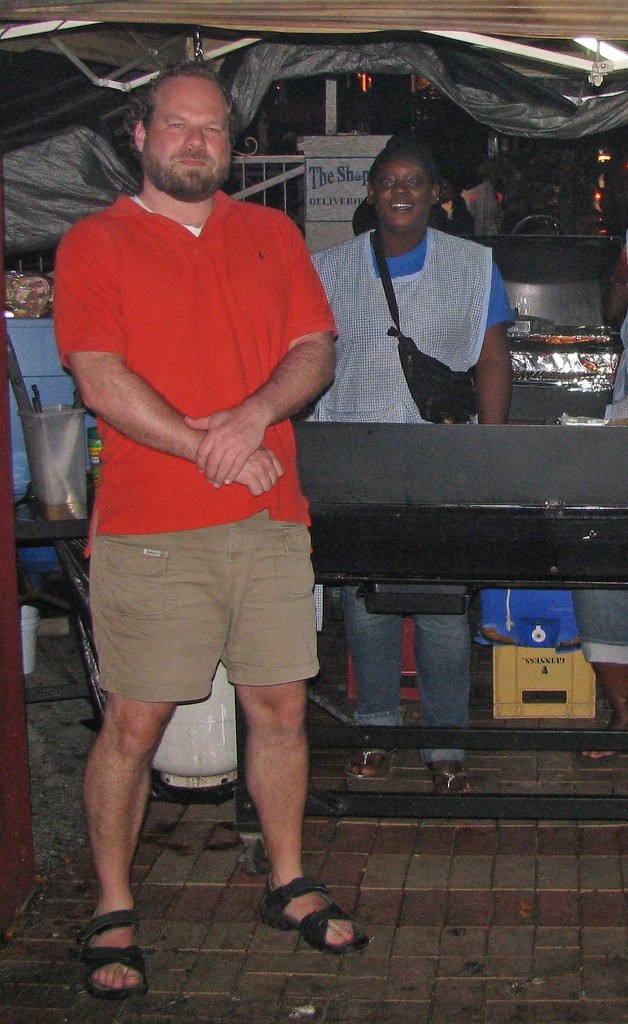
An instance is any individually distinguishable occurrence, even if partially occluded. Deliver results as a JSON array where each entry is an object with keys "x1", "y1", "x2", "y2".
[
  {"x1": 295, "y1": 138, "x2": 516, "y2": 794},
  {"x1": 479, "y1": 585, "x2": 581, "y2": 654},
  {"x1": 571, "y1": 229, "x2": 628, "y2": 763},
  {"x1": 53, "y1": 63, "x2": 370, "y2": 1001}
]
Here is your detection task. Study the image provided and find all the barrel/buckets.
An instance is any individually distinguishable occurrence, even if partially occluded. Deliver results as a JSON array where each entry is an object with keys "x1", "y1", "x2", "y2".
[{"x1": 21, "y1": 606, "x2": 41, "y2": 675}]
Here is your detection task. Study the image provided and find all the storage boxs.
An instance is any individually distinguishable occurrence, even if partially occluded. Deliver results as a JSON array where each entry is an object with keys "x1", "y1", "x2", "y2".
[
  {"x1": 7, "y1": 319, "x2": 100, "y2": 496},
  {"x1": 491, "y1": 644, "x2": 597, "y2": 720}
]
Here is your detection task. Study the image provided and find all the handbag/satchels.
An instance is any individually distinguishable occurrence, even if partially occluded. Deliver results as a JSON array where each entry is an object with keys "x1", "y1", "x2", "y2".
[{"x1": 371, "y1": 228, "x2": 480, "y2": 424}]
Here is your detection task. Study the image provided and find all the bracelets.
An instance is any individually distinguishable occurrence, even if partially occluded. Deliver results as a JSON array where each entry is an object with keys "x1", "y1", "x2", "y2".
[{"x1": 607, "y1": 273, "x2": 628, "y2": 288}]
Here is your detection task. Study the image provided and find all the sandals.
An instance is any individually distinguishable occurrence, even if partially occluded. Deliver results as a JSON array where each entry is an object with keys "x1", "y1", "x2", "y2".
[
  {"x1": 259, "y1": 872, "x2": 369, "y2": 953},
  {"x1": 80, "y1": 910, "x2": 150, "y2": 1001},
  {"x1": 426, "y1": 759, "x2": 469, "y2": 795},
  {"x1": 342, "y1": 747, "x2": 387, "y2": 778}
]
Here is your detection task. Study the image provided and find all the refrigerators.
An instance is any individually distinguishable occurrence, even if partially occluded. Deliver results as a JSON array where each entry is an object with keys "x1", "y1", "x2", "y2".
[{"x1": 6, "y1": 319, "x2": 77, "y2": 494}]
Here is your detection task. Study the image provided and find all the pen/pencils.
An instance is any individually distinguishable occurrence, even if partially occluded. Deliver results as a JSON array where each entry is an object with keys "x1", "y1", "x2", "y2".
[{"x1": 259, "y1": 252, "x2": 264, "y2": 260}]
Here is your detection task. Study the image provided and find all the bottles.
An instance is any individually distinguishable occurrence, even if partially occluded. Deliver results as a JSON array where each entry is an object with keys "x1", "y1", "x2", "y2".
[{"x1": 88, "y1": 426, "x2": 102, "y2": 495}]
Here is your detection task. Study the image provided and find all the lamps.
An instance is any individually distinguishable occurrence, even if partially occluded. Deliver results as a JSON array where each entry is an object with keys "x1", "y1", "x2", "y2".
[{"x1": 595, "y1": 146, "x2": 612, "y2": 162}]
